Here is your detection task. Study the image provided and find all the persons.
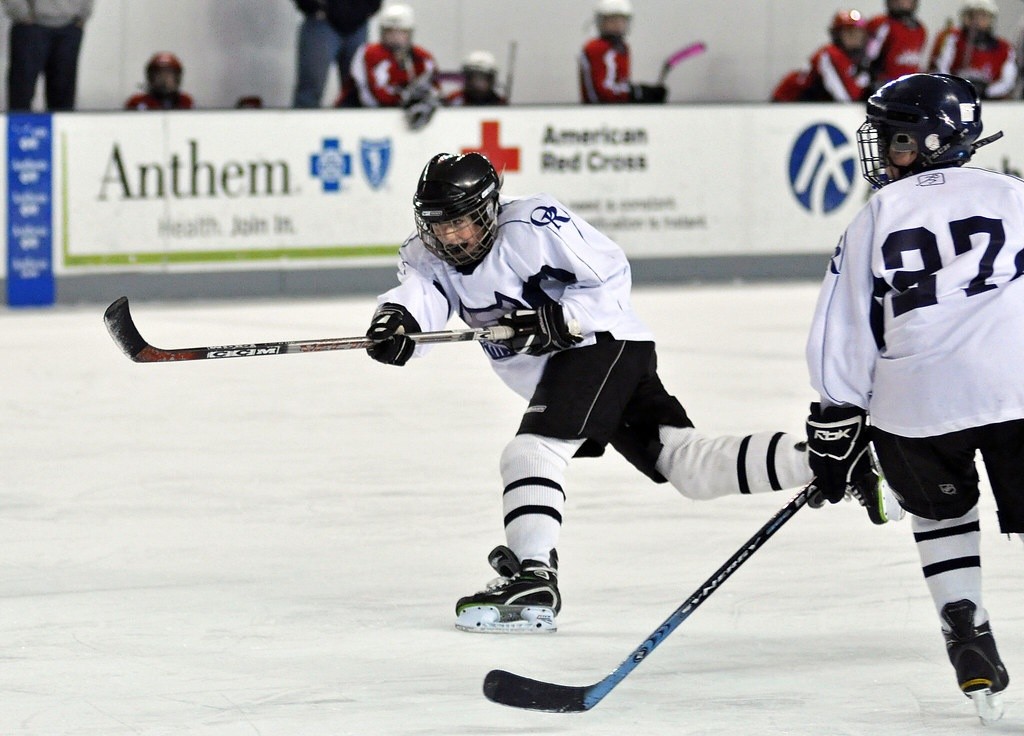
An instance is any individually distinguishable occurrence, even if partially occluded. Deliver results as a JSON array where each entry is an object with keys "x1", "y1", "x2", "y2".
[
  {"x1": 802, "y1": 11, "x2": 869, "y2": 101},
  {"x1": 805, "y1": 74, "x2": 1024, "y2": 727},
  {"x1": 864, "y1": 0, "x2": 925, "y2": 101},
  {"x1": 292, "y1": 0, "x2": 382, "y2": 108},
  {"x1": 367, "y1": 152, "x2": 906, "y2": 634},
  {"x1": 348, "y1": 7, "x2": 507, "y2": 128},
  {"x1": 929, "y1": 0, "x2": 1018, "y2": 100},
  {"x1": 577, "y1": 0, "x2": 668, "y2": 104},
  {"x1": 125, "y1": 52, "x2": 193, "y2": 109},
  {"x1": 0, "y1": 0, "x2": 94, "y2": 112}
]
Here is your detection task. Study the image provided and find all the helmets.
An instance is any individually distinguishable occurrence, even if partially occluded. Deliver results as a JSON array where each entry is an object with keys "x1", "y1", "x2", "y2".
[
  {"x1": 592, "y1": 1, "x2": 634, "y2": 23},
  {"x1": 962, "y1": 1, "x2": 998, "y2": 29},
  {"x1": 830, "y1": 13, "x2": 863, "y2": 31},
  {"x1": 145, "y1": 50, "x2": 184, "y2": 88},
  {"x1": 461, "y1": 53, "x2": 497, "y2": 80},
  {"x1": 855, "y1": 73, "x2": 982, "y2": 192},
  {"x1": 413, "y1": 154, "x2": 500, "y2": 267},
  {"x1": 379, "y1": 5, "x2": 414, "y2": 33}
]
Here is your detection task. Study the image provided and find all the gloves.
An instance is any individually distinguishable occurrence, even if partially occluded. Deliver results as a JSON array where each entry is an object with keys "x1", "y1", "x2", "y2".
[
  {"x1": 482, "y1": 292, "x2": 582, "y2": 359},
  {"x1": 366, "y1": 302, "x2": 417, "y2": 366},
  {"x1": 803, "y1": 401, "x2": 866, "y2": 505}
]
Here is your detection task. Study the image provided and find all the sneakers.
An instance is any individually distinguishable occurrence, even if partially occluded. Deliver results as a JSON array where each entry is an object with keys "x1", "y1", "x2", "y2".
[
  {"x1": 841, "y1": 443, "x2": 904, "y2": 526},
  {"x1": 451, "y1": 545, "x2": 561, "y2": 631},
  {"x1": 937, "y1": 600, "x2": 1013, "y2": 725}
]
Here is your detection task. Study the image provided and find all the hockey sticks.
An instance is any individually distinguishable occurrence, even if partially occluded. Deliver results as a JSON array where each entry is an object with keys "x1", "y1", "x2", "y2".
[
  {"x1": 502, "y1": 40, "x2": 517, "y2": 97},
  {"x1": 103, "y1": 296, "x2": 516, "y2": 364},
  {"x1": 656, "y1": 40, "x2": 708, "y2": 82},
  {"x1": 484, "y1": 475, "x2": 823, "y2": 715}
]
